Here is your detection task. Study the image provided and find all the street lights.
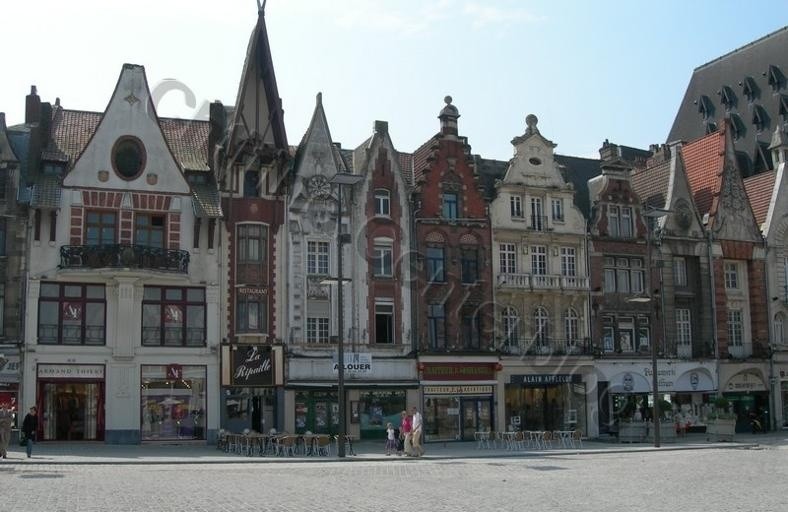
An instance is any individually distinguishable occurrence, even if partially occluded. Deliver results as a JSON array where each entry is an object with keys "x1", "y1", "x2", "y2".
[
  {"x1": 639, "y1": 207, "x2": 675, "y2": 448},
  {"x1": 327, "y1": 172, "x2": 363, "y2": 457}
]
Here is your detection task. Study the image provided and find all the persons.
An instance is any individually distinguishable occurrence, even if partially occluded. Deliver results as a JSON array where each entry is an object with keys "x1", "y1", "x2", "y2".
[
  {"x1": 21, "y1": 404, "x2": 40, "y2": 458},
  {"x1": 408, "y1": 406, "x2": 426, "y2": 457},
  {"x1": 1, "y1": 402, "x2": 13, "y2": 459},
  {"x1": 398, "y1": 411, "x2": 414, "y2": 457},
  {"x1": 384, "y1": 422, "x2": 397, "y2": 457}
]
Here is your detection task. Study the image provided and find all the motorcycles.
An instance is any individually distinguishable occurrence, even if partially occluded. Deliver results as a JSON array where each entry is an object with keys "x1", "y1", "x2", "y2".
[{"x1": 750, "y1": 411, "x2": 768, "y2": 434}]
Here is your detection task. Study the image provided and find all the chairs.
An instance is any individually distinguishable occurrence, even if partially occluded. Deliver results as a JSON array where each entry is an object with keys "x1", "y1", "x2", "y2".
[
  {"x1": 474, "y1": 431, "x2": 584, "y2": 451},
  {"x1": 217, "y1": 433, "x2": 357, "y2": 458}
]
